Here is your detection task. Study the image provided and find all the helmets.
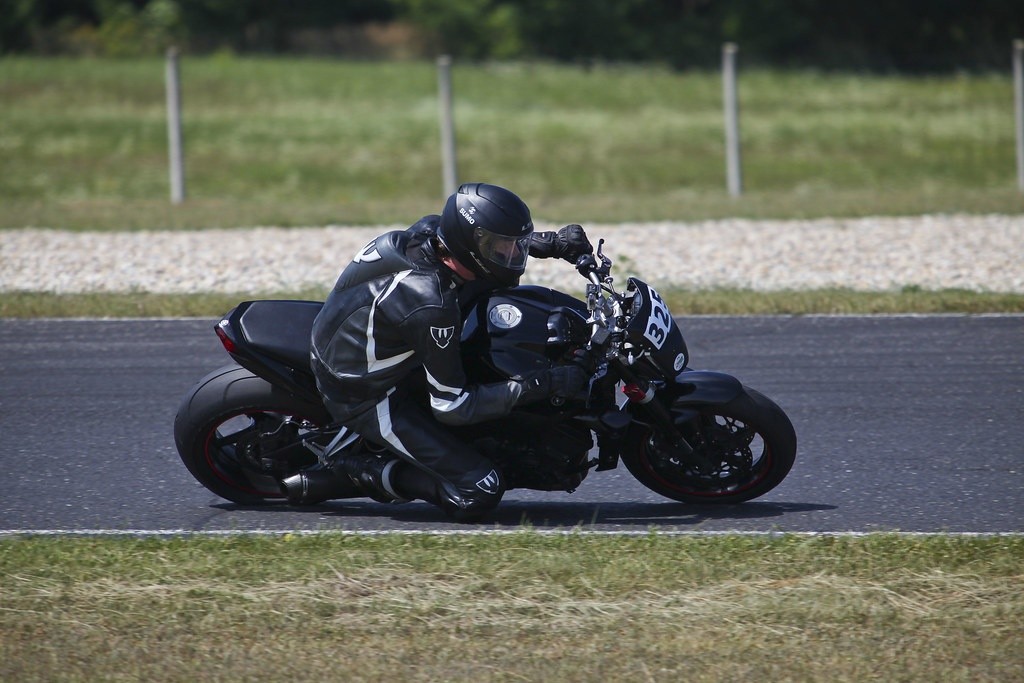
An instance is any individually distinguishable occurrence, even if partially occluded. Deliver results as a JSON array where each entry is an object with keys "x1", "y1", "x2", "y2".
[{"x1": 441, "y1": 182, "x2": 533, "y2": 289}]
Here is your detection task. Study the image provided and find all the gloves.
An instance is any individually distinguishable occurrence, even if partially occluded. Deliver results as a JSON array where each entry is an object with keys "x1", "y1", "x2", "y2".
[
  {"x1": 536, "y1": 366, "x2": 589, "y2": 402},
  {"x1": 555, "y1": 223, "x2": 593, "y2": 264}
]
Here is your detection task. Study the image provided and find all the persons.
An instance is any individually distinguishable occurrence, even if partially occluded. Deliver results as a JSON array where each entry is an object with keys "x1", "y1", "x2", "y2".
[{"x1": 311, "y1": 182, "x2": 594, "y2": 519}]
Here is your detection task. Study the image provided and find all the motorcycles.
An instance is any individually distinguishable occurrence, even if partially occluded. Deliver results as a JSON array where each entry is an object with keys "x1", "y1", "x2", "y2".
[{"x1": 172, "y1": 225, "x2": 797, "y2": 506}]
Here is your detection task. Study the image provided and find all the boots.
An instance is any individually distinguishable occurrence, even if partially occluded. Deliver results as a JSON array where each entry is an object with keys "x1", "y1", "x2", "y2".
[{"x1": 345, "y1": 451, "x2": 413, "y2": 506}]
